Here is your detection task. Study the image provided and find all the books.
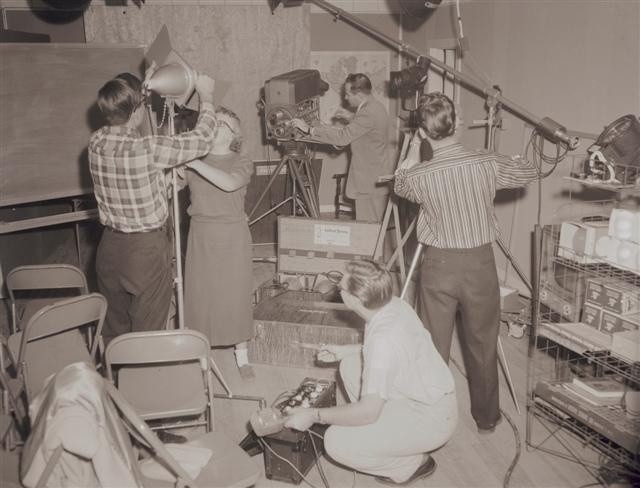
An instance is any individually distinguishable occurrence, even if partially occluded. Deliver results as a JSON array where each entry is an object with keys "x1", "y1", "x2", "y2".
[{"x1": 538, "y1": 318, "x2": 639, "y2": 438}]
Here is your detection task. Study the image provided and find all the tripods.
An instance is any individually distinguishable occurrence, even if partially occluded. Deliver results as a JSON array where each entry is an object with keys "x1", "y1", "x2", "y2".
[{"x1": 241, "y1": 143, "x2": 320, "y2": 226}]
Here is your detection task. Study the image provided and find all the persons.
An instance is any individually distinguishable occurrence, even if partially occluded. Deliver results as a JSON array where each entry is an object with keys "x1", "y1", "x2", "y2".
[
  {"x1": 167, "y1": 104, "x2": 260, "y2": 385},
  {"x1": 83, "y1": 71, "x2": 218, "y2": 460},
  {"x1": 392, "y1": 93, "x2": 543, "y2": 437},
  {"x1": 289, "y1": 70, "x2": 396, "y2": 221},
  {"x1": 281, "y1": 257, "x2": 459, "y2": 487}
]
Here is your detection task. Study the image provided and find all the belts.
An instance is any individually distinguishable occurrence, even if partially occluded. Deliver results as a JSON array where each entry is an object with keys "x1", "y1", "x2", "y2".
[{"x1": 106, "y1": 225, "x2": 163, "y2": 235}]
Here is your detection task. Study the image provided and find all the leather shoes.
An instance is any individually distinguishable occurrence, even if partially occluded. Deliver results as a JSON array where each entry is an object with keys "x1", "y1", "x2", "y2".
[
  {"x1": 162, "y1": 432, "x2": 188, "y2": 444},
  {"x1": 478, "y1": 413, "x2": 502, "y2": 434},
  {"x1": 375, "y1": 454, "x2": 437, "y2": 488}
]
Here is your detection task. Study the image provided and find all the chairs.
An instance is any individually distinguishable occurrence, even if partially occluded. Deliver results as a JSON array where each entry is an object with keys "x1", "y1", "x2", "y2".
[
  {"x1": 108, "y1": 326, "x2": 217, "y2": 438},
  {"x1": 5, "y1": 294, "x2": 108, "y2": 444},
  {"x1": 7, "y1": 264, "x2": 93, "y2": 325}
]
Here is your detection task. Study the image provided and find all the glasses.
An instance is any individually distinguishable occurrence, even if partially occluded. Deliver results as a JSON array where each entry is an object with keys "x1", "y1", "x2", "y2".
[{"x1": 217, "y1": 120, "x2": 235, "y2": 135}]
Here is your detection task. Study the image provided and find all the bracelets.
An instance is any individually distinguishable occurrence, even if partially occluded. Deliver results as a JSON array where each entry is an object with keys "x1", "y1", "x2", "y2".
[
  {"x1": 308, "y1": 125, "x2": 314, "y2": 135},
  {"x1": 316, "y1": 407, "x2": 324, "y2": 424},
  {"x1": 409, "y1": 142, "x2": 421, "y2": 146}
]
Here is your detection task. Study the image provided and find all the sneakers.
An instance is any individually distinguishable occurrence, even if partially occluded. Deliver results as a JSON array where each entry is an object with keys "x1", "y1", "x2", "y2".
[{"x1": 238, "y1": 364, "x2": 254, "y2": 383}]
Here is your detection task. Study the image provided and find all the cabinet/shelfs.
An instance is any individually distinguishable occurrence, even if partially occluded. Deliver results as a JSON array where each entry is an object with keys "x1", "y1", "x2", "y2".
[{"x1": 522, "y1": 151, "x2": 640, "y2": 474}]
